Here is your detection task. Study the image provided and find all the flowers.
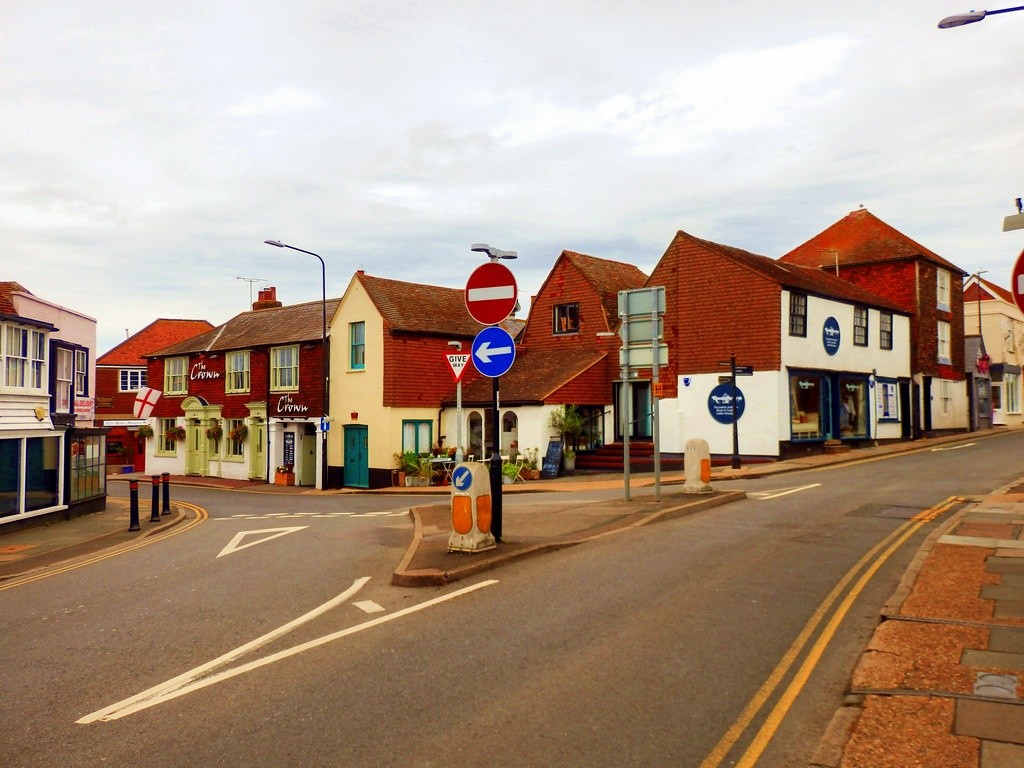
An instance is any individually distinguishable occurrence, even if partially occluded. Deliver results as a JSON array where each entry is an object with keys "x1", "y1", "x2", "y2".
[
  {"x1": 525, "y1": 446, "x2": 539, "y2": 470},
  {"x1": 277, "y1": 466, "x2": 289, "y2": 473},
  {"x1": 229, "y1": 425, "x2": 248, "y2": 443},
  {"x1": 137, "y1": 424, "x2": 154, "y2": 438},
  {"x1": 205, "y1": 426, "x2": 222, "y2": 439},
  {"x1": 165, "y1": 426, "x2": 186, "y2": 441}
]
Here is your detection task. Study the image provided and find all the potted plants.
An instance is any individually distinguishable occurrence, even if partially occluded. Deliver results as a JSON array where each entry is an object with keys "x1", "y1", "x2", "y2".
[
  {"x1": 563, "y1": 450, "x2": 576, "y2": 470},
  {"x1": 393, "y1": 451, "x2": 439, "y2": 488}
]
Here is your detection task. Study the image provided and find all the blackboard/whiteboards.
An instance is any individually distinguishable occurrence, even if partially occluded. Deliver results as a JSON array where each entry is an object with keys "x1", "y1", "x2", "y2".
[{"x1": 542, "y1": 441, "x2": 564, "y2": 479}]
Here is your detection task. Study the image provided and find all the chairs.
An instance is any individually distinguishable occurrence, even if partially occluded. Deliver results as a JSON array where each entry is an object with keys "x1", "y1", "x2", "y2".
[{"x1": 429, "y1": 453, "x2": 526, "y2": 485}]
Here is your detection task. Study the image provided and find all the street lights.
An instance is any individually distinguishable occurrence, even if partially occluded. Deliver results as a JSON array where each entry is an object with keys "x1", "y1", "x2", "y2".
[
  {"x1": 976, "y1": 270, "x2": 989, "y2": 335},
  {"x1": 266, "y1": 239, "x2": 327, "y2": 491}
]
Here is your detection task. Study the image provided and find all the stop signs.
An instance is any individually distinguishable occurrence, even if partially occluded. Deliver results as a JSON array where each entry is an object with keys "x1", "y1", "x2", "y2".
[
  {"x1": 465, "y1": 263, "x2": 518, "y2": 327},
  {"x1": 1011, "y1": 248, "x2": 1024, "y2": 313}
]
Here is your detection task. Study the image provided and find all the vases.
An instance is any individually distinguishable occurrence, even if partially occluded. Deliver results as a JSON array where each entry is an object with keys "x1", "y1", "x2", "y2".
[
  {"x1": 275, "y1": 472, "x2": 295, "y2": 486},
  {"x1": 521, "y1": 468, "x2": 540, "y2": 480}
]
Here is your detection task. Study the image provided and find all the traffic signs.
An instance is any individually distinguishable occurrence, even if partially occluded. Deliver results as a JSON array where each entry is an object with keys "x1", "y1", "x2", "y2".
[{"x1": 717, "y1": 361, "x2": 755, "y2": 377}]
